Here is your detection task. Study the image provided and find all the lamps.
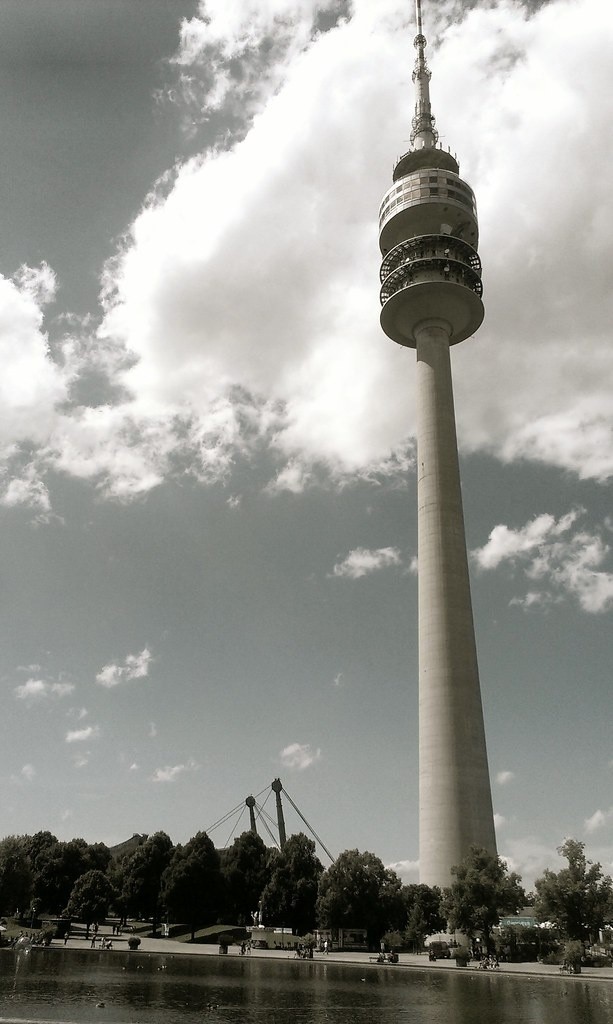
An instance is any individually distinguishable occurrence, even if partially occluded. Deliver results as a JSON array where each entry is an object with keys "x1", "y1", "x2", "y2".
[{"x1": 565, "y1": 941, "x2": 583, "y2": 974}]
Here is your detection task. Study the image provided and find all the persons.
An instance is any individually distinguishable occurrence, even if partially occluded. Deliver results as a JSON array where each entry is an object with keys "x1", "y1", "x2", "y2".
[
  {"x1": 377, "y1": 949, "x2": 396, "y2": 963},
  {"x1": 90, "y1": 935, "x2": 113, "y2": 949},
  {"x1": 91, "y1": 920, "x2": 136, "y2": 936},
  {"x1": 449, "y1": 939, "x2": 512, "y2": 969},
  {"x1": 19, "y1": 930, "x2": 70, "y2": 946},
  {"x1": 323, "y1": 940, "x2": 329, "y2": 955},
  {"x1": 240, "y1": 940, "x2": 252, "y2": 955}
]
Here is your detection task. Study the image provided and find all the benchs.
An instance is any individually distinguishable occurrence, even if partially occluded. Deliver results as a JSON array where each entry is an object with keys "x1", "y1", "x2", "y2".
[
  {"x1": 479, "y1": 963, "x2": 494, "y2": 968},
  {"x1": 369, "y1": 957, "x2": 388, "y2": 962}
]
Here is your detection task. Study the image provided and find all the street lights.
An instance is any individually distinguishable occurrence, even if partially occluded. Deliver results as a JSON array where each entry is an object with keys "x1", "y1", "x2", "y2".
[{"x1": 31, "y1": 907, "x2": 37, "y2": 929}]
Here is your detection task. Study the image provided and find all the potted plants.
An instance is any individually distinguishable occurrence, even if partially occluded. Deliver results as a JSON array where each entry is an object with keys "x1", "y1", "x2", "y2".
[
  {"x1": 382, "y1": 933, "x2": 404, "y2": 963},
  {"x1": 451, "y1": 946, "x2": 469, "y2": 966},
  {"x1": 40, "y1": 923, "x2": 57, "y2": 943},
  {"x1": 128, "y1": 937, "x2": 141, "y2": 950},
  {"x1": 218, "y1": 934, "x2": 233, "y2": 954},
  {"x1": 99, "y1": 945, "x2": 113, "y2": 950}
]
